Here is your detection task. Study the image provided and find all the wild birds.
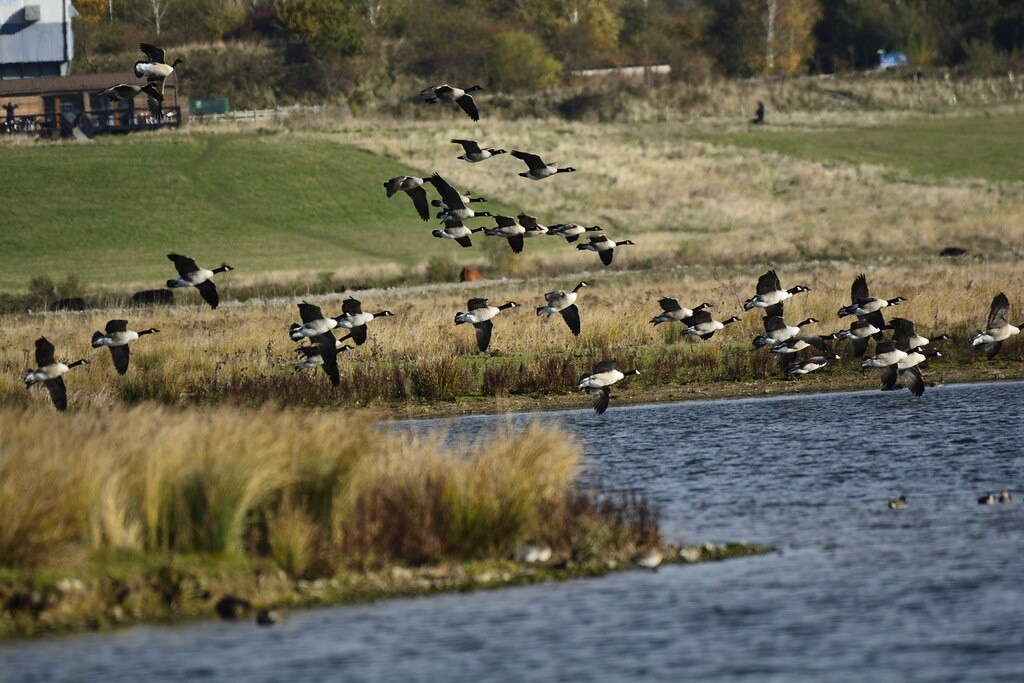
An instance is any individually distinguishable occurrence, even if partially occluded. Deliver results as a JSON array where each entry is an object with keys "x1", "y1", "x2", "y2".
[
  {"x1": 382, "y1": 171, "x2": 636, "y2": 268},
  {"x1": 95, "y1": 42, "x2": 187, "y2": 121},
  {"x1": 23, "y1": 319, "x2": 162, "y2": 413},
  {"x1": 536, "y1": 281, "x2": 594, "y2": 338},
  {"x1": 576, "y1": 360, "x2": 647, "y2": 416},
  {"x1": 420, "y1": 84, "x2": 486, "y2": 123},
  {"x1": 449, "y1": 137, "x2": 578, "y2": 180},
  {"x1": 887, "y1": 492, "x2": 911, "y2": 512},
  {"x1": 454, "y1": 296, "x2": 524, "y2": 351},
  {"x1": 743, "y1": 269, "x2": 955, "y2": 397},
  {"x1": 164, "y1": 249, "x2": 237, "y2": 310},
  {"x1": 287, "y1": 295, "x2": 396, "y2": 388},
  {"x1": 977, "y1": 489, "x2": 1014, "y2": 506},
  {"x1": 648, "y1": 294, "x2": 742, "y2": 342},
  {"x1": 968, "y1": 291, "x2": 1024, "y2": 361}
]
